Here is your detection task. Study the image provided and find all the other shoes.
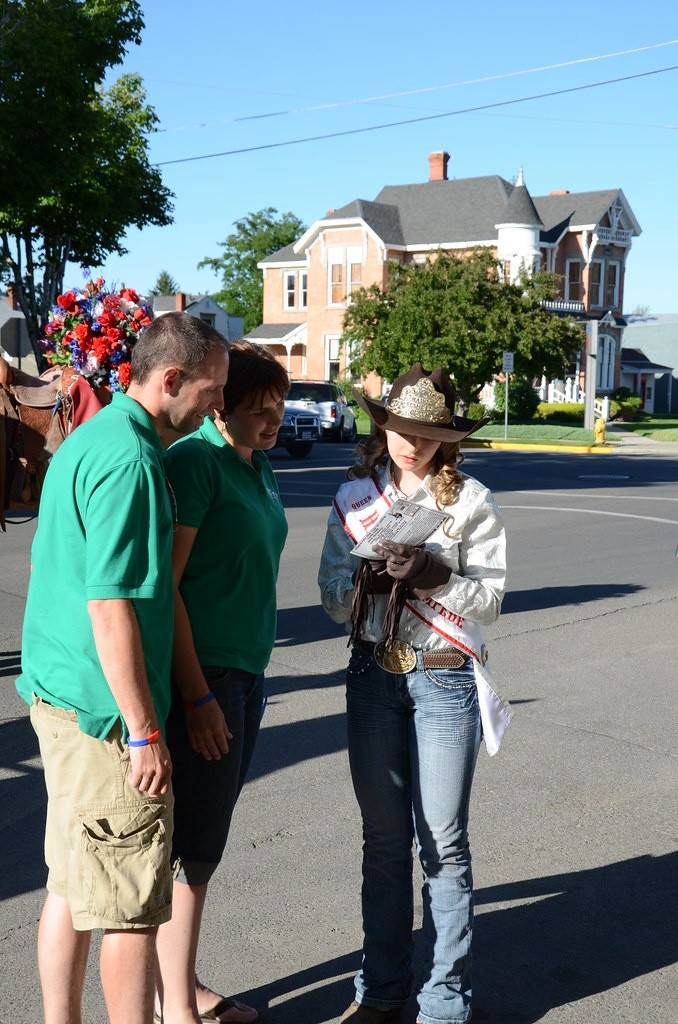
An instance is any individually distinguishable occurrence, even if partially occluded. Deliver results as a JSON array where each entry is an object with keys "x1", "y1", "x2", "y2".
[{"x1": 336, "y1": 1001, "x2": 402, "y2": 1023}]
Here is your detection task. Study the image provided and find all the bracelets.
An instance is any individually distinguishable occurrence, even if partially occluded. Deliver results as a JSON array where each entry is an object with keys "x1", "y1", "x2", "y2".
[
  {"x1": 183, "y1": 691, "x2": 214, "y2": 711},
  {"x1": 127, "y1": 729, "x2": 162, "y2": 746}
]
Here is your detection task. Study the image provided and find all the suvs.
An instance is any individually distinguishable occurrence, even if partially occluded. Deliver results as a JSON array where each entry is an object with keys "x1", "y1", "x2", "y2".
[
  {"x1": 284, "y1": 379, "x2": 358, "y2": 443},
  {"x1": 263, "y1": 407, "x2": 323, "y2": 456}
]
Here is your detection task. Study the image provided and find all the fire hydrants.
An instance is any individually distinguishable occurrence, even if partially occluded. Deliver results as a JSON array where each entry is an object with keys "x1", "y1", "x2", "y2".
[{"x1": 595, "y1": 418, "x2": 606, "y2": 444}]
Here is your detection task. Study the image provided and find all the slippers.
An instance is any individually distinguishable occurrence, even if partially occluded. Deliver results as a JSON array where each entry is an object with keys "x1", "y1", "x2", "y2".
[{"x1": 153, "y1": 983, "x2": 262, "y2": 1024}]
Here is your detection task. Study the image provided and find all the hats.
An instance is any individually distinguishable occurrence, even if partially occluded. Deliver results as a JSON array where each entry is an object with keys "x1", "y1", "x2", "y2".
[{"x1": 351, "y1": 361, "x2": 492, "y2": 443}]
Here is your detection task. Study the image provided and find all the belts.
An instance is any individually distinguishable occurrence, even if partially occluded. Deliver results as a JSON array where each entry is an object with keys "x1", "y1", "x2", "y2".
[{"x1": 353, "y1": 639, "x2": 469, "y2": 675}]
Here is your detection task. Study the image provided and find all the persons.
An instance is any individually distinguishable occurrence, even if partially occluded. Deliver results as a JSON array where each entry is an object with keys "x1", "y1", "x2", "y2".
[
  {"x1": 318, "y1": 362, "x2": 515, "y2": 1024},
  {"x1": 153, "y1": 340, "x2": 290, "y2": 1024},
  {"x1": 13, "y1": 311, "x2": 230, "y2": 1024}
]
